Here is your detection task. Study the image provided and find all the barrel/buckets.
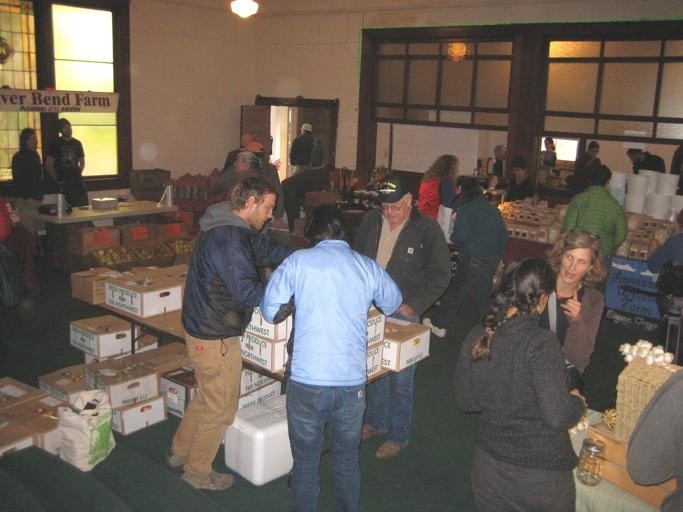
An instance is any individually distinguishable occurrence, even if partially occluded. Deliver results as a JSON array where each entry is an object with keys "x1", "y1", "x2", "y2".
[{"x1": 606, "y1": 169, "x2": 683, "y2": 219}]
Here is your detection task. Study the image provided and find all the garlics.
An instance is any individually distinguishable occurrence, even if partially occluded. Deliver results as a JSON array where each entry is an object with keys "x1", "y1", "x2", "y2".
[{"x1": 618, "y1": 339, "x2": 675, "y2": 367}]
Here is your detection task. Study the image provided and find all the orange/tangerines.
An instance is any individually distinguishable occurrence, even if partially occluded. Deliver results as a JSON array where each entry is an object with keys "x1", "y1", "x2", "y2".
[{"x1": 95, "y1": 239, "x2": 194, "y2": 267}]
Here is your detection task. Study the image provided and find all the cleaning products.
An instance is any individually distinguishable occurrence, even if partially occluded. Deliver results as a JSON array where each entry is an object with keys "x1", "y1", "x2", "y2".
[
  {"x1": 173, "y1": 168, "x2": 222, "y2": 201},
  {"x1": 371, "y1": 167, "x2": 381, "y2": 183}
]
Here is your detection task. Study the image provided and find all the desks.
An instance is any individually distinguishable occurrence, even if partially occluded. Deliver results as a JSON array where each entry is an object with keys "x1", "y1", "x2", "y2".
[
  {"x1": 29, "y1": 200, "x2": 177, "y2": 225},
  {"x1": 587, "y1": 407, "x2": 683, "y2": 512}
]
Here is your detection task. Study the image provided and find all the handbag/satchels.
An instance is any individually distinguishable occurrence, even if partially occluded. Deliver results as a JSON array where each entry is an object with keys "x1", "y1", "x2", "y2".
[
  {"x1": 57, "y1": 391, "x2": 115, "y2": 472},
  {"x1": 437, "y1": 203, "x2": 458, "y2": 244},
  {"x1": 657, "y1": 262, "x2": 683, "y2": 315}
]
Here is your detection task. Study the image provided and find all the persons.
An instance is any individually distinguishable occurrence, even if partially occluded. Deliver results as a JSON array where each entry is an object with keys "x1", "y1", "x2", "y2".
[
  {"x1": 504, "y1": 159, "x2": 533, "y2": 202},
  {"x1": 417, "y1": 154, "x2": 507, "y2": 339},
  {"x1": 353, "y1": 176, "x2": 451, "y2": 460},
  {"x1": 539, "y1": 229, "x2": 606, "y2": 390},
  {"x1": 562, "y1": 165, "x2": 626, "y2": 292},
  {"x1": 204, "y1": 123, "x2": 331, "y2": 206},
  {"x1": 166, "y1": 177, "x2": 301, "y2": 491},
  {"x1": 575, "y1": 142, "x2": 600, "y2": 184},
  {"x1": 452, "y1": 258, "x2": 588, "y2": 512},
  {"x1": 43, "y1": 116, "x2": 90, "y2": 205},
  {"x1": 8, "y1": 127, "x2": 46, "y2": 202},
  {"x1": 625, "y1": 369, "x2": 682, "y2": 512},
  {"x1": 0, "y1": 194, "x2": 41, "y2": 311},
  {"x1": 260, "y1": 204, "x2": 403, "y2": 511},
  {"x1": 647, "y1": 210, "x2": 683, "y2": 339},
  {"x1": 627, "y1": 148, "x2": 664, "y2": 173},
  {"x1": 539, "y1": 137, "x2": 556, "y2": 170}
]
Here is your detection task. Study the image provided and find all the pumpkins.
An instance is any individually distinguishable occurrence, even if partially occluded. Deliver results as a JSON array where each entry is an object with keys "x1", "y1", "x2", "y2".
[{"x1": 601, "y1": 408, "x2": 616, "y2": 430}]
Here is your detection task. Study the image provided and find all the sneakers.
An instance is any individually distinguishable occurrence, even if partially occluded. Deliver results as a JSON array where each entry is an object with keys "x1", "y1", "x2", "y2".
[
  {"x1": 361, "y1": 422, "x2": 408, "y2": 460},
  {"x1": 165, "y1": 448, "x2": 234, "y2": 490},
  {"x1": 422, "y1": 317, "x2": 446, "y2": 338}
]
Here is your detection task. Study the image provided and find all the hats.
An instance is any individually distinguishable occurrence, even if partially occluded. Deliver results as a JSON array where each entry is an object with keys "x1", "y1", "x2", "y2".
[
  {"x1": 300, "y1": 123, "x2": 313, "y2": 132},
  {"x1": 376, "y1": 173, "x2": 408, "y2": 203},
  {"x1": 240, "y1": 141, "x2": 265, "y2": 154}
]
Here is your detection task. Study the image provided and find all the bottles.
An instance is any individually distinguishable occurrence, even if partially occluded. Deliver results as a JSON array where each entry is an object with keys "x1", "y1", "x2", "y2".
[{"x1": 576, "y1": 437, "x2": 608, "y2": 486}]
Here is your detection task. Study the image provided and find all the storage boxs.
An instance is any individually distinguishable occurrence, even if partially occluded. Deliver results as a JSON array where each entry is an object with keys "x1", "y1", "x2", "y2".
[
  {"x1": 59, "y1": 224, "x2": 430, "y2": 396},
  {"x1": 1, "y1": 361, "x2": 293, "y2": 487}
]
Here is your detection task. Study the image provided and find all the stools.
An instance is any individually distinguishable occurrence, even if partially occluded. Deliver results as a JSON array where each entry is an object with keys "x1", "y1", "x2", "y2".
[{"x1": 663, "y1": 315, "x2": 682, "y2": 365}]
